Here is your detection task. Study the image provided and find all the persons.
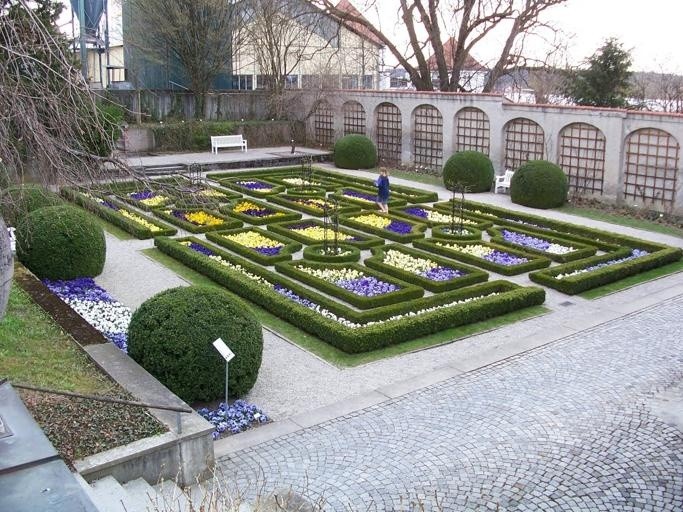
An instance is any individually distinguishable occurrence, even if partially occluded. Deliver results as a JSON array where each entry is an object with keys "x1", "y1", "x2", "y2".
[{"x1": 374, "y1": 167, "x2": 389, "y2": 214}]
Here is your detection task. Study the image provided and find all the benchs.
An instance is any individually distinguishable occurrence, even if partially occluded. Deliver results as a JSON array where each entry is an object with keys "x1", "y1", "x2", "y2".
[
  {"x1": 210, "y1": 134, "x2": 248, "y2": 154},
  {"x1": 494, "y1": 171, "x2": 514, "y2": 194}
]
